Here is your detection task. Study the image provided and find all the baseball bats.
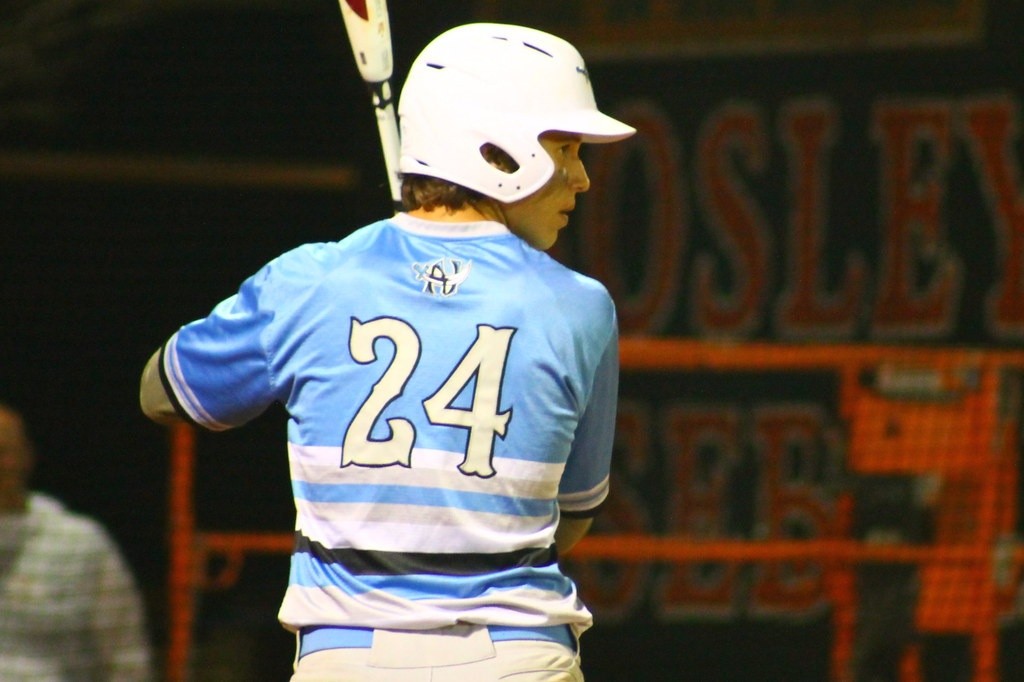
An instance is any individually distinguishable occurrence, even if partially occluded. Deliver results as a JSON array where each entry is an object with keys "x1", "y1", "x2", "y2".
[{"x1": 337, "y1": 0, "x2": 406, "y2": 215}]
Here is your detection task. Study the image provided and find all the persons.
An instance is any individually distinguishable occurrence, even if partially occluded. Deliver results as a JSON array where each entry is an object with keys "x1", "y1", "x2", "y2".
[
  {"x1": 142, "y1": 22, "x2": 637, "y2": 682},
  {"x1": 1, "y1": 404, "x2": 158, "y2": 682}
]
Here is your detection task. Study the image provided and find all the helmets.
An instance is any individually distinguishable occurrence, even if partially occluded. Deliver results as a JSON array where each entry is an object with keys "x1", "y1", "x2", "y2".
[{"x1": 396, "y1": 22, "x2": 639, "y2": 204}]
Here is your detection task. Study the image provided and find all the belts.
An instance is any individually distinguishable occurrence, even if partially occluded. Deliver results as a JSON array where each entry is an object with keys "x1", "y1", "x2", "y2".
[{"x1": 300, "y1": 623, "x2": 579, "y2": 660}]
